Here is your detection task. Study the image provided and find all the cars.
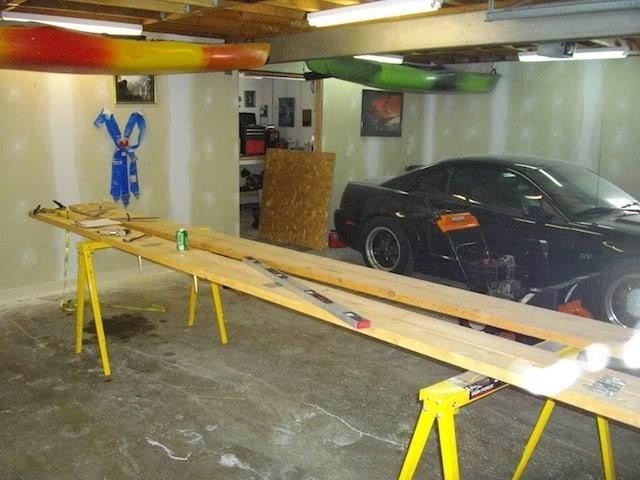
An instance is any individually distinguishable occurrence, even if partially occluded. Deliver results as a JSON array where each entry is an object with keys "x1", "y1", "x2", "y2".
[{"x1": 334, "y1": 154, "x2": 640, "y2": 327}]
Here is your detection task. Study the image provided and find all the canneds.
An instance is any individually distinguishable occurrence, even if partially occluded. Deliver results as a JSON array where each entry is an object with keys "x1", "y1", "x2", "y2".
[{"x1": 176, "y1": 229, "x2": 188, "y2": 251}]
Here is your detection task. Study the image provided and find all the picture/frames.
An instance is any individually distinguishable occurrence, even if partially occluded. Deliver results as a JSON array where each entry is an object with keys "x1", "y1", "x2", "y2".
[
  {"x1": 359, "y1": 90, "x2": 404, "y2": 138},
  {"x1": 110, "y1": 72, "x2": 157, "y2": 105},
  {"x1": 278, "y1": 97, "x2": 296, "y2": 127},
  {"x1": 243, "y1": 90, "x2": 257, "y2": 108}
]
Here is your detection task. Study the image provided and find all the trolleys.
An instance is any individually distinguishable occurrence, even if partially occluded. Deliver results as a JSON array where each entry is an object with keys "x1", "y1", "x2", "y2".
[{"x1": 408, "y1": 188, "x2": 603, "y2": 321}]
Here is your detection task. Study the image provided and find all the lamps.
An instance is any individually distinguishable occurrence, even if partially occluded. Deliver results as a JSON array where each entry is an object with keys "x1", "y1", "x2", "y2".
[
  {"x1": 306, "y1": 0, "x2": 443, "y2": 28},
  {"x1": 517, "y1": 46, "x2": 628, "y2": 63},
  {"x1": 351, "y1": 54, "x2": 405, "y2": 65}
]
